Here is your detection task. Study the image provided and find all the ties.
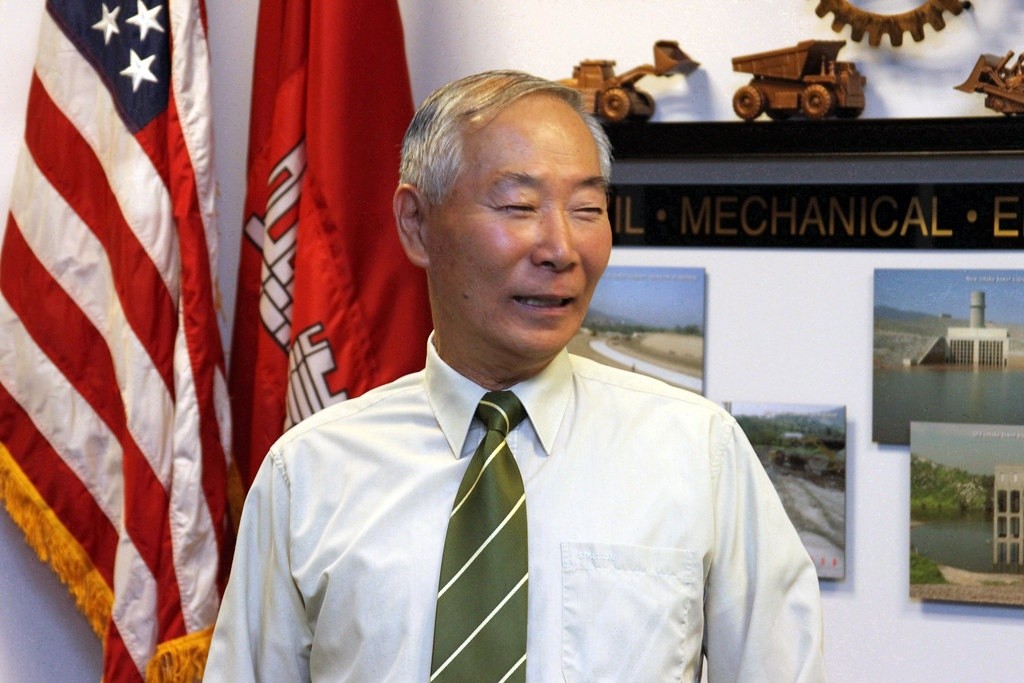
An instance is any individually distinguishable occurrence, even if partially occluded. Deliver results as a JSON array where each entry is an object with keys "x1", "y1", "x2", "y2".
[{"x1": 430, "y1": 391, "x2": 528, "y2": 683}]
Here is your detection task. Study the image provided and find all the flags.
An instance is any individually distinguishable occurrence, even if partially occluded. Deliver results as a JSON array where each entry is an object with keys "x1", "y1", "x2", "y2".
[
  {"x1": 229, "y1": 0, "x2": 434, "y2": 532},
  {"x1": 0, "y1": 0, "x2": 233, "y2": 683}
]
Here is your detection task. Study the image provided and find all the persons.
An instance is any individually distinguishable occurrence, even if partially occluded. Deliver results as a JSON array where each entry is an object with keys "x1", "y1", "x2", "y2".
[{"x1": 203, "y1": 71, "x2": 826, "y2": 683}]
